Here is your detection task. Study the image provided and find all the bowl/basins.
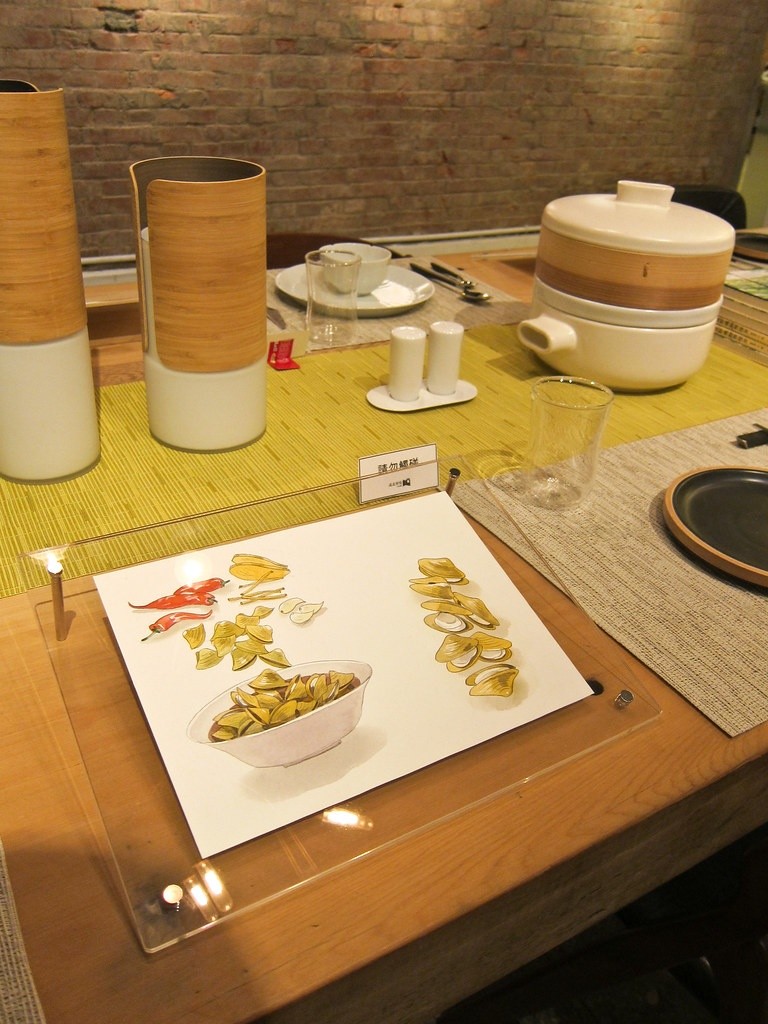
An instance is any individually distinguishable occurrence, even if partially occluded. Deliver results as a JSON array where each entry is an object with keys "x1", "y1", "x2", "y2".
[{"x1": 317, "y1": 241, "x2": 393, "y2": 297}]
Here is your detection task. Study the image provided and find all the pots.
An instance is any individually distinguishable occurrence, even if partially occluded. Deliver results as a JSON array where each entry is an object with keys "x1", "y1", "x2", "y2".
[{"x1": 516, "y1": 180, "x2": 738, "y2": 393}]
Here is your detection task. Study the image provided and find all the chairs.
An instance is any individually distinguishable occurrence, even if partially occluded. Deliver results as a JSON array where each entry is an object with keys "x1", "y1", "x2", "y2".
[{"x1": 673, "y1": 184, "x2": 748, "y2": 231}]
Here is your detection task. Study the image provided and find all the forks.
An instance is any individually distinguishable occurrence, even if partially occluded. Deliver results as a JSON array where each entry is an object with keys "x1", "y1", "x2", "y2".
[{"x1": 409, "y1": 263, "x2": 480, "y2": 288}]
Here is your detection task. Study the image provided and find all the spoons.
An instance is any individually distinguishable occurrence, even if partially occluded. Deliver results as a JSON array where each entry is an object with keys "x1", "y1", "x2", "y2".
[{"x1": 431, "y1": 263, "x2": 490, "y2": 300}]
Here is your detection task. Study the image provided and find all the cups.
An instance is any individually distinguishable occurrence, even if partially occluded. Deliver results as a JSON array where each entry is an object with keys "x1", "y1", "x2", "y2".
[
  {"x1": 523, "y1": 377, "x2": 611, "y2": 507},
  {"x1": 304, "y1": 249, "x2": 361, "y2": 346}
]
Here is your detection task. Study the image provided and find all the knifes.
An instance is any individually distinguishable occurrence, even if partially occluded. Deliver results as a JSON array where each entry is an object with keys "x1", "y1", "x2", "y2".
[{"x1": 266, "y1": 305, "x2": 286, "y2": 330}]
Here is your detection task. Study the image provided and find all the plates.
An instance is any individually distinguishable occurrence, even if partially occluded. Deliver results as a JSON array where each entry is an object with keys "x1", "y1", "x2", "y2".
[
  {"x1": 664, "y1": 463, "x2": 768, "y2": 588},
  {"x1": 274, "y1": 261, "x2": 435, "y2": 318}
]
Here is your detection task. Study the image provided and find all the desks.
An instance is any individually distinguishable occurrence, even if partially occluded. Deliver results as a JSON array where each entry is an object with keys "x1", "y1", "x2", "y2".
[{"x1": 0, "y1": 246, "x2": 768, "y2": 1024}]
